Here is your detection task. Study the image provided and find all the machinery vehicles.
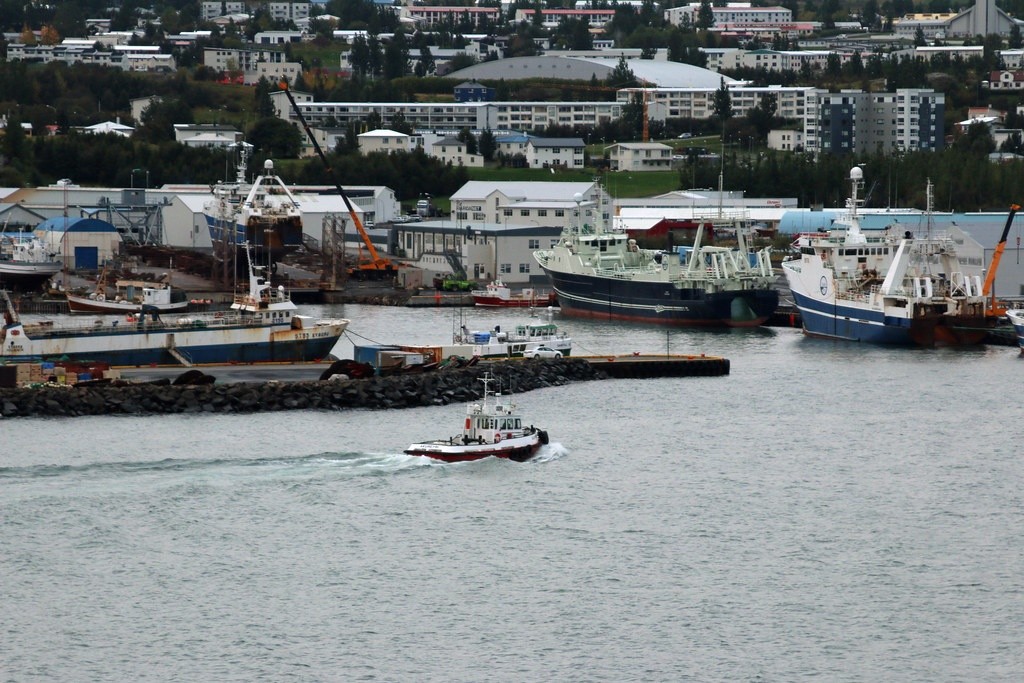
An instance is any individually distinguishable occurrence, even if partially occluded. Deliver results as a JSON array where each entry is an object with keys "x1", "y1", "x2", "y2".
[
  {"x1": 276, "y1": 75, "x2": 398, "y2": 281},
  {"x1": 432, "y1": 250, "x2": 477, "y2": 292},
  {"x1": 983, "y1": 204, "x2": 1021, "y2": 316}
]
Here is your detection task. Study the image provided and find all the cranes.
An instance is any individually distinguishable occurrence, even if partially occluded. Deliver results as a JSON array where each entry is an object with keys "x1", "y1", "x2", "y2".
[{"x1": 530, "y1": 78, "x2": 657, "y2": 142}]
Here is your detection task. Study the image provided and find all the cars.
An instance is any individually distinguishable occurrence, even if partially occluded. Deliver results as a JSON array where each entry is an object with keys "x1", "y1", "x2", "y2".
[
  {"x1": 409, "y1": 214, "x2": 422, "y2": 222},
  {"x1": 388, "y1": 217, "x2": 404, "y2": 223},
  {"x1": 678, "y1": 133, "x2": 691, "y2": 138},
  {"x1": 523, "y1": 346, "x2": 563, "y2": 360}
]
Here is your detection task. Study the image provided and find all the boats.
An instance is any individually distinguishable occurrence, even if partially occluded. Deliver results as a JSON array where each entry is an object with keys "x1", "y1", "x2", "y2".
[
  {"x1": 532, "y1": 184, "x2": 780, "y2": 328},
  {"x1": 67, "y1": 257, "x2": 188, "y2": 316},
  {"x1": 202, "y1": 149, "x2": 303, "y2": 264},
  {"x1": 1005, "y1": 309, "x2": 1024, "y2": 354},
  {"x1": 781, "y1": 166, "x2": 999, "y2": 346},
  {"x1": 403, "y1": 371, "x2": 549, "y2": 463},
  {"x1": 0, "y1": 212, "x2": 64, "y2": 293},
  {"x1": 0, "y1": 240, "x2": 351, "y2": 366},
  {"x1": 451, "y1": 306, "x2": 572, "y2": 356},
  {"x1": 49, "y1": 280, "x2": 90, "y2": 298},
  {"x1": 471, "y1": 277, "x2": 549, "y2": 309}
]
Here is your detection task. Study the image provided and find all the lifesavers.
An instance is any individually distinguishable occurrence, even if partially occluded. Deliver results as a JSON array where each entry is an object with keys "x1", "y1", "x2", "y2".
[
  {"x1": 821, "y1": 253, "x2": 825, "y2": 259},
  {"x1": 494, "y1": 433, "x2": 501, "y2": 444}
]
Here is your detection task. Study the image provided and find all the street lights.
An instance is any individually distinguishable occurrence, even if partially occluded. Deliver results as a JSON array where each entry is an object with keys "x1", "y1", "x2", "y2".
[
  {"x1": 601, "y1": 138, "x2": 605, "y2": 155},
  {"x1": 588, "y1": 133, "x2": 591, "y2": 144},
  {"x1": 530, "y1": 219, "x2": 540, "y2": 234}
]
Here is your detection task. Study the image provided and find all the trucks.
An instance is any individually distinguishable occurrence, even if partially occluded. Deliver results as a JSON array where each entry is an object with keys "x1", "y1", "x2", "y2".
[{"x1": 417, "y1": 200, "x2": 442, "y2": 217}]
